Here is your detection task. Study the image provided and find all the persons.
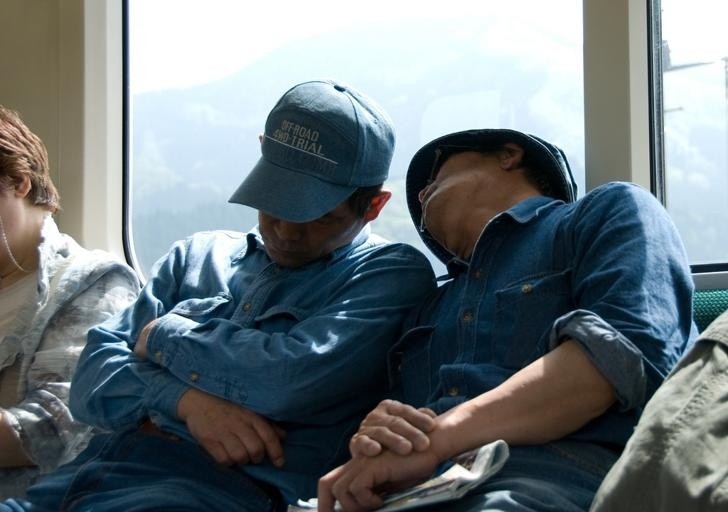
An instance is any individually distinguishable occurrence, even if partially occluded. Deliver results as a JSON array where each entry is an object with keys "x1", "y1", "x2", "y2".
[
  {"x1": 318, "y1": 129, "x2": 700, "y2": 512},
  {"x1": 588, "y1": 308, "x2": 728, "y2": 512},
  {"x1": 0, "y1": 79, "x2": 436, "y2": 512},
  {"x1": 0, "y1": 107, "x2": 142, "y2": 504}
]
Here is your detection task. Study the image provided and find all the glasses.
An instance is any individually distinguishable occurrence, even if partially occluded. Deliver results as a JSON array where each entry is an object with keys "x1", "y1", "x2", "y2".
[{"x1": 418, "y1": 143, "x2": 504, "y2": 242}]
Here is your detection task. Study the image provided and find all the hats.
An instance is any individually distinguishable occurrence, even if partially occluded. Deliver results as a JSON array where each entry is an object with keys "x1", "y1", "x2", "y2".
[
  {"x1": 229, "y1": 81, "x2": 394, "y2": 225},
  {"x1": 406, "y1": 129, "x2": 577, "y2": 263}
]
